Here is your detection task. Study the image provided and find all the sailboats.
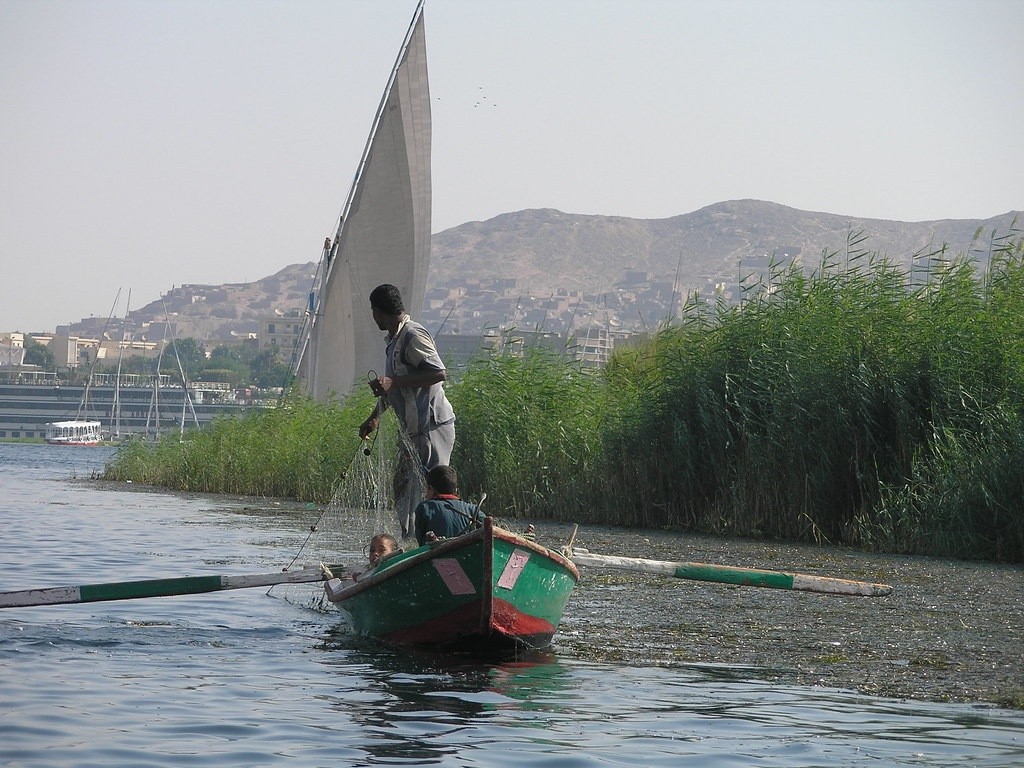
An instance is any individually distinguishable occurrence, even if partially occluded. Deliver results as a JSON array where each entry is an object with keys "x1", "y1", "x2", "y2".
[{"x1": 44, "y1": 287, "x2": 122, "y2": 446}]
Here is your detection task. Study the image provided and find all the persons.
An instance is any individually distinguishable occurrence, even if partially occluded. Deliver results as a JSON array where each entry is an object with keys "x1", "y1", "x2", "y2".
[
  {"x1": 359, "y1": 284, "x2": 456, "y2": 541},
  {"x1": 415, "y1": 465, "x2": 486, "y2": 547},
  {"x1": 369, "y1": 534, "x2": 397, "y2": 564}
]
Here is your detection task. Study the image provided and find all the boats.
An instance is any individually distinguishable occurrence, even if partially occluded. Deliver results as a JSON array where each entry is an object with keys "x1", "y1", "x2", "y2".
[
  {"x1": 0, "y1": 363, "x2": 279, "y2": 436},
  {"x1": 323, "y1": 516, "x2": 582, "y2": 653}
]
